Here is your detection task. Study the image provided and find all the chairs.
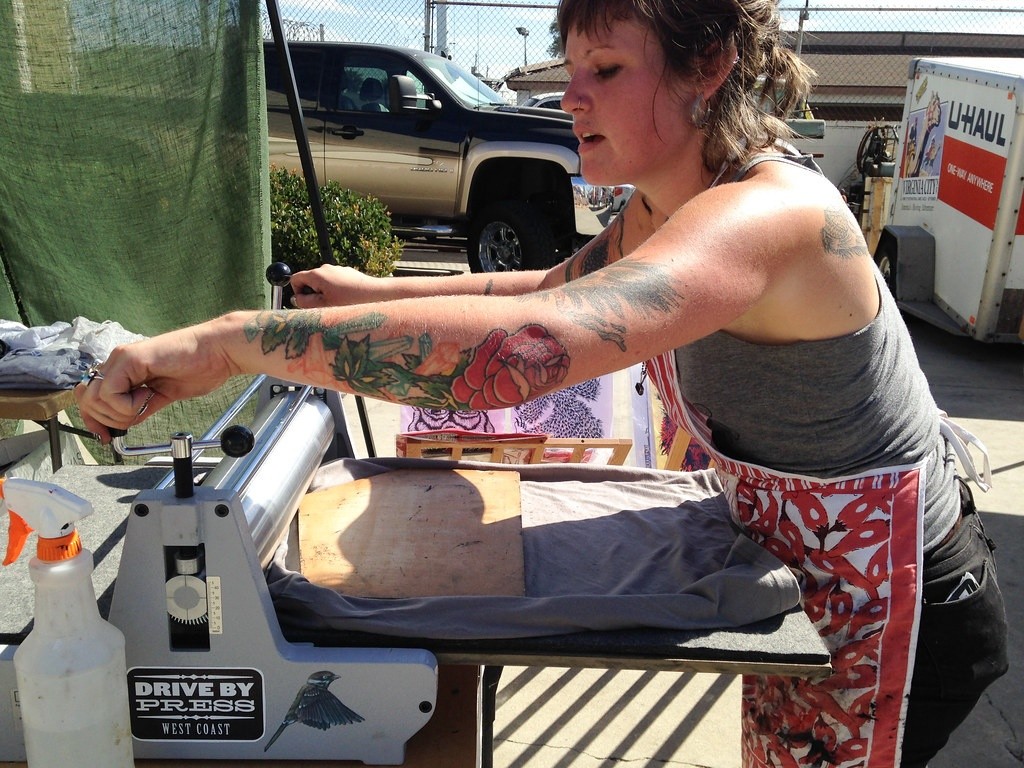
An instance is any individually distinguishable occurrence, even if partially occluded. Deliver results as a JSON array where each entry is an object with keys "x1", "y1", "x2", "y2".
[
  {"x1": 360, "y1": 78, "x2": 389, "y2": 112},
  {"x1": 339, "y1": 70, "x2": 356, "y2": 110}
]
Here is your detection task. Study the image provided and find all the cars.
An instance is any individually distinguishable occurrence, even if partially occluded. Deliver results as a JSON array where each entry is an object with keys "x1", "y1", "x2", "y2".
[
  {"x1": 752, "y1": 77, "x2": 815, "y2": 120},
  {"x1": 521, "y1": 90, "x2": 567, "y2": 111}
]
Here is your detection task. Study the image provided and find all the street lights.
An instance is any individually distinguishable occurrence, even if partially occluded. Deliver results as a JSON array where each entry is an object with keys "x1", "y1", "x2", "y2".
[{"x1": 516, "y1": 27, "x2": 530, "y2": 76}]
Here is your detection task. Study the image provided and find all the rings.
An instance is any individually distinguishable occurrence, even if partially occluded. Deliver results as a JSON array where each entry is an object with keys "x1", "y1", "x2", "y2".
[{"x1": 86, "y1": 366, "x2": 105, "y2": 380}]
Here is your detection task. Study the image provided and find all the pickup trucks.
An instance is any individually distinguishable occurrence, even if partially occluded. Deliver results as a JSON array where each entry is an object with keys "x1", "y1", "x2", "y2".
[{"x1": 0, "y1": 39, "x2": 637, "y2": 281}]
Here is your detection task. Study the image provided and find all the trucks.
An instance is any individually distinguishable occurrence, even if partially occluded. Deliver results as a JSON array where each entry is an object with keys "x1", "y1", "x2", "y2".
[{"x1": 879, "y1": 56, "x2": 1024, "y2": 347}]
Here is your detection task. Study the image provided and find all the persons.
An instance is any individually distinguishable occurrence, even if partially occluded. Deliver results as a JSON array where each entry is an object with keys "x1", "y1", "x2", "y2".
[{"x1": 73, "y1": 0, "x2": 1009, "y2": 768}]
